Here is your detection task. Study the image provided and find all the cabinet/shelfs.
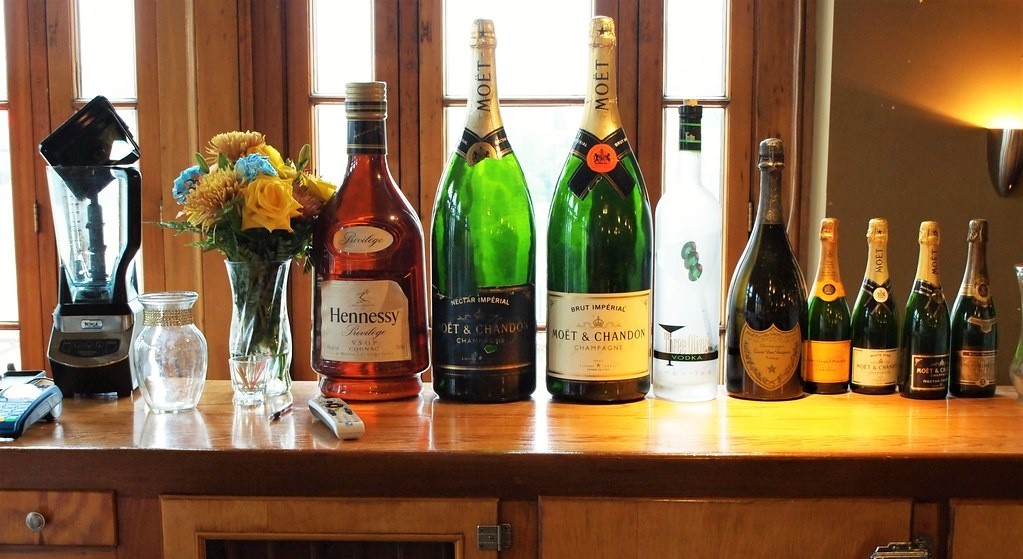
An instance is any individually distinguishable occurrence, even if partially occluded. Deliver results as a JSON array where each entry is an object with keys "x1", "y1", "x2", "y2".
[{"x1": 0, "y1": 374, "x2": 1022, "y2": 559}]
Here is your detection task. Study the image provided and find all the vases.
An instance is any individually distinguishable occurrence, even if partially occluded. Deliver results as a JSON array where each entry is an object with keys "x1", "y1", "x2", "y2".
[{"x1": 220, "y1": 255, "x2": 294, "y2": 399}]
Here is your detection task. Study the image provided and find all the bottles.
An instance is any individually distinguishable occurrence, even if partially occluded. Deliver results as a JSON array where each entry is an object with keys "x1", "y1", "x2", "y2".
[
  {"x1": 431, "y1": 22, "x2": 537, "y2": 403},
  {"x1": 849, "y1": 218, "x2": 900, "y2": 394},
  {"x1": 653, "y1": 98, "x2": 722, "y2": 401},
  {"x1": 951, "y1": 219, "x2": 999, "y2": 398},
  {"x1": 800, "y1": 217, "x2": 851, "y2": 394},
  {"x1": 134, "y1": 291, "x2": 209, "y2": 413},
  {"x1": 310, "y1": 84, "x2": 431, "y2": 401},
  {"x1": 724, "y1": 138, "x2": 806, "y2": 401},
  {"x1": 899, "y1": 221, "x2": 951, "y2": 400},
  {"x1": 540, "y1": 16, "x2": 656, "y2": 405}
]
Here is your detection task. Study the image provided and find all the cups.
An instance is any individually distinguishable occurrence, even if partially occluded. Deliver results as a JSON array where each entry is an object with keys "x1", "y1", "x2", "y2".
[{"x1": 229, "y1": 355, "x2": 267, "y2": 405}]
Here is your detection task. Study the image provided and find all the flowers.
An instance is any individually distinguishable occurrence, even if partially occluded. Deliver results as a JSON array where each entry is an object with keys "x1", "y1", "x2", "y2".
[{"x1": 136, "y1": 125, "x2": 336, "y2": 379}]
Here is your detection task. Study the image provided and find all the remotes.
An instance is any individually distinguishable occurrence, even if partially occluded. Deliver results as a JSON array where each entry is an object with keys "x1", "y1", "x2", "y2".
[{"x1": 308, "y1": 396, "x2": 367, "y2": 443}]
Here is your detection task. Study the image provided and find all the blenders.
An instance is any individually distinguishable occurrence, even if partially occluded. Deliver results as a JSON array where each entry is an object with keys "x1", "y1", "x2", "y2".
[{"x1": 36, "y1": 95, "x2": 141, "y2": 399}]
